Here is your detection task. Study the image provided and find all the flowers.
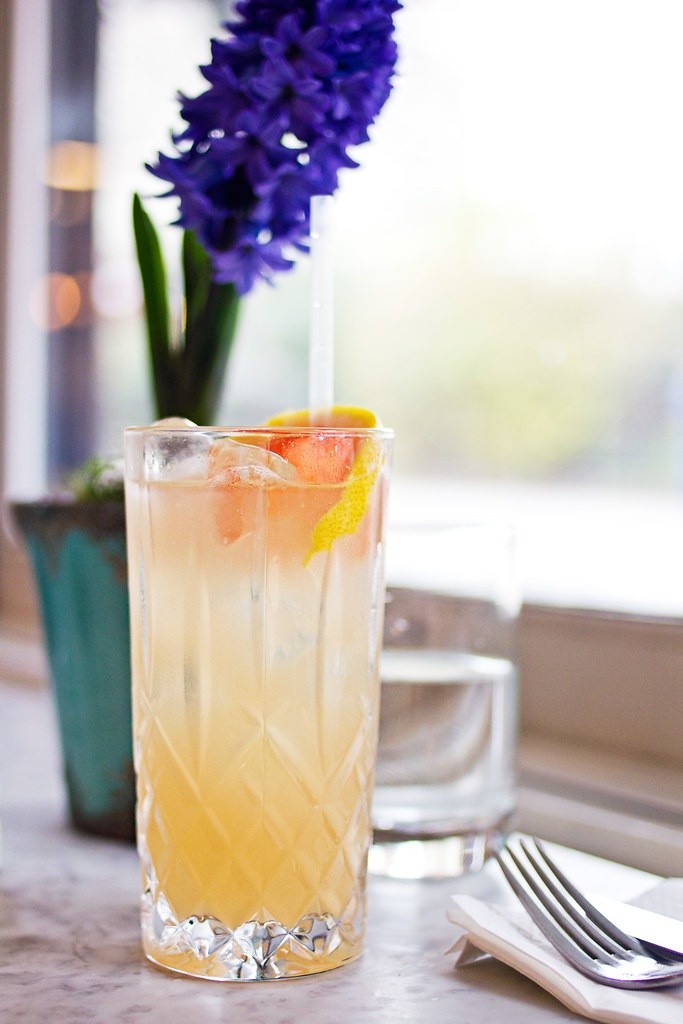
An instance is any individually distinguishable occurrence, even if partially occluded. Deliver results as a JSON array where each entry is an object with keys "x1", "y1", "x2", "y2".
[{"x1": 131, "y1": 0, "x2": 403, "y2": 420}]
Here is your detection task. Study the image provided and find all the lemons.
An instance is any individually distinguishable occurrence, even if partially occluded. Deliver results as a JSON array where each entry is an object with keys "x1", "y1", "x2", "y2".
[{"x1": 233, "y1": 403, "x2": 381, "y2": 557}]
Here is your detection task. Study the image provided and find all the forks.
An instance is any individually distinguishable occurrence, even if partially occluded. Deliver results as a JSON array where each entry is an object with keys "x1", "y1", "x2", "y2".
[{"x1": 491, "y1": 834, "x2": 683, "y2": 991}]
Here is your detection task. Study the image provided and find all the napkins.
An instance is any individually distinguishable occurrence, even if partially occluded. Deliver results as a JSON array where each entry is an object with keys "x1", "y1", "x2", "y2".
[{"x1": 440, "y1": 840, "x2": 682, "y2": 1024}]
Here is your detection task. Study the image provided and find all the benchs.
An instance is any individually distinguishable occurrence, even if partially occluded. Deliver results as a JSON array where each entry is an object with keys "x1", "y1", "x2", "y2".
[{"x1": 378, "y1": 587, "x2": 683, "y2": 874}]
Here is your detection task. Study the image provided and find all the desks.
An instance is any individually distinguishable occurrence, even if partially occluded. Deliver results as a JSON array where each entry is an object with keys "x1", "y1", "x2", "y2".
[{"x1": 0, "y1": 690, "x2": 683, "y2": 1024}]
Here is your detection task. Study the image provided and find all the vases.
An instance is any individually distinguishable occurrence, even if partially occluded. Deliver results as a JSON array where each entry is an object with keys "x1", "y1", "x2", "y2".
[{"x1": 10, "y1": 488, "x2": 136, "y2": 842}]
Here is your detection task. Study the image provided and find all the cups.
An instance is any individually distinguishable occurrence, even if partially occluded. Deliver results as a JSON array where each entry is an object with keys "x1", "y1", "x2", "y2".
[
  {"x1": 121, "y1": 424, "x2": 398, "y2": 983},
  {"x1": 366, "y1": 516, "x2": 523, "y2": 880}
]
